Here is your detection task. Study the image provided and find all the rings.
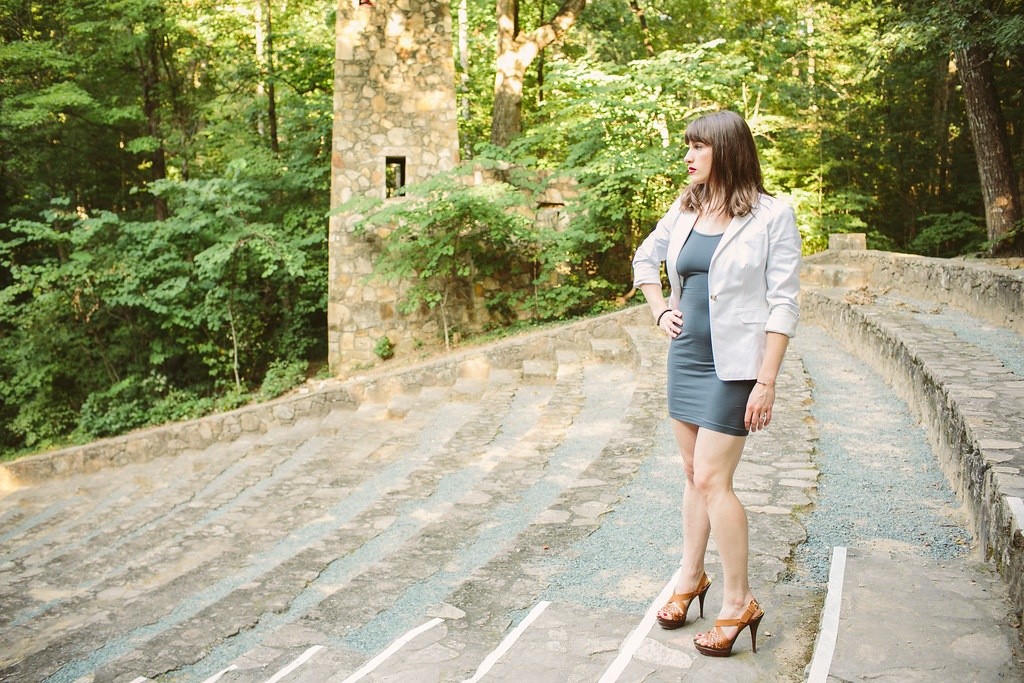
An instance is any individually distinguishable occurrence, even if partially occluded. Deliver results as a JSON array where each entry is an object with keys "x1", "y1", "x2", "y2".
[{"x1": 760, "y1": 416, "x2": 765, "y2": 419}]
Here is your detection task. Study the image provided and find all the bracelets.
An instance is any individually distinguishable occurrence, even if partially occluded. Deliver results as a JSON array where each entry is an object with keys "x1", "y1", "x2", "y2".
[
  {"x1": 657, "y1": 309, "x2": 672, "y2": 325},
  {"x1": 757, "y1": 381, "x2": 777, "y2": 386}
]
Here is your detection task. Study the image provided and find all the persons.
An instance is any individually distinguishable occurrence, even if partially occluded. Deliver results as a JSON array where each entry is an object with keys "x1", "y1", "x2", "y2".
[{"x1": 632, "y1": 111, "x2": 802, "y2": 657}]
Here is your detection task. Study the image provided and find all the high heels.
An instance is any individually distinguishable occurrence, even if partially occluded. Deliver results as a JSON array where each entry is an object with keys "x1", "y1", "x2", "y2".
[
  {"x1": 693, "y1": 599, "x2": 765, "y2": 657},
  {"x1": 657, "y1": 571, "x2": 712, "y2": 630}
]
